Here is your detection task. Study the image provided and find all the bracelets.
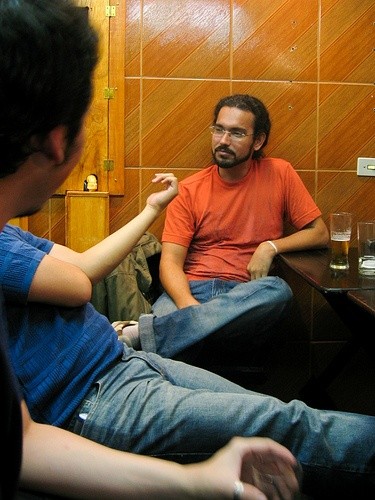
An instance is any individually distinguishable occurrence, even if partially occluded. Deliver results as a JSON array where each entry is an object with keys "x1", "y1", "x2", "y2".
[{"x1": 267, "y1": 240, "x2": 278, "y2": 255}]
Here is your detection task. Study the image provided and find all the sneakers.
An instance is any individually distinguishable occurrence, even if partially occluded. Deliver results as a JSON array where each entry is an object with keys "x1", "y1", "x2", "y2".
[{"x1": 110, "y1": 319, "x2": 138, "y2": 347}]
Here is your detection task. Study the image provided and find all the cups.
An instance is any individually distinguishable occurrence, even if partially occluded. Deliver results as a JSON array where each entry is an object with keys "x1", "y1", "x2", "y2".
[
  {"x1": 330, "y1": 213, "x2": 352, "y2": 269},
  {"x1": 357, "y1": 222, "x2": 375, "y2": 275}
]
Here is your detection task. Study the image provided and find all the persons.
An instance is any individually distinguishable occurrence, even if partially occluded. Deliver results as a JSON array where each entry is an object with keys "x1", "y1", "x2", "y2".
[
  {"x1": 111, "y1": 94, "x2": 329, "y2": 373},
  {"x1": 0, "y1": 173, "x2": 375, "y2": 500},
  {"x1": 0, "y1": 0, "x2": 300, "y2": 500}
]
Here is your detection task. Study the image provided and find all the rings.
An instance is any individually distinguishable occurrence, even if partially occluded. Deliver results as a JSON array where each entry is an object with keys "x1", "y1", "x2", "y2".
[{"x1": 233, "y1": 480, "x2": 244, "y2": 500}]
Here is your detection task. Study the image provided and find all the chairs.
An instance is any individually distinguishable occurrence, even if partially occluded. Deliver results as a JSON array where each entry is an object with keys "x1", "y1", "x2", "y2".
[{"x1": 92, "y1": 231, "x2": 163, "y2": 325}]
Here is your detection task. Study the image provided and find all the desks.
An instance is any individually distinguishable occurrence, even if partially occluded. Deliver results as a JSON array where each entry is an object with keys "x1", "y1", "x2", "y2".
[{"x1": 279, "y1": 247, "x2": 374, "y2": 409}]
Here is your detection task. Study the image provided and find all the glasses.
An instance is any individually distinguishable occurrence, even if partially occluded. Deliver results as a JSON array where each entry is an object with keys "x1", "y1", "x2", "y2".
[{"x1": 209, "y1": 124, "x2": 259, "y2": 139}]
